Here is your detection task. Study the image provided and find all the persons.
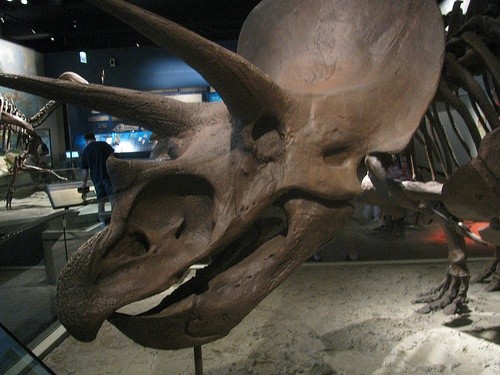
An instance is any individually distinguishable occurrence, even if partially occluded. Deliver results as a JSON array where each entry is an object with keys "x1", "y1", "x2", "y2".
[
  {"x1": 27, "y1": 133, "x2": 51, "y2": 198},
  {"x1": 76, "y1": 132, "x2": 115, "y2": 227},
  {"x1": 371, "y1": 152, "x2": 409, "y2": 237}
]
